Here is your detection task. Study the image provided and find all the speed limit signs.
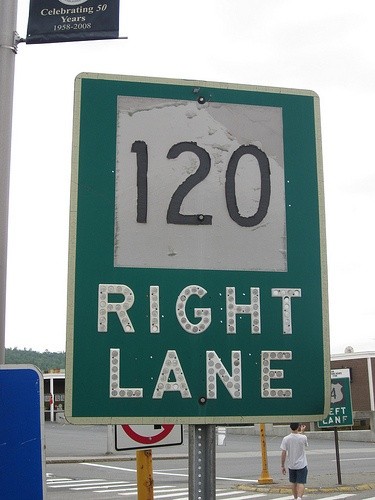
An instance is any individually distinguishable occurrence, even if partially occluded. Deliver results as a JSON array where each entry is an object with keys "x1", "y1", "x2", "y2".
[
  {"x1": 63, "y1": 72, "x2": 331, "y2": 423},
  {"x1": 317, "y1": 368, "x2": 354, "y2": 429}
]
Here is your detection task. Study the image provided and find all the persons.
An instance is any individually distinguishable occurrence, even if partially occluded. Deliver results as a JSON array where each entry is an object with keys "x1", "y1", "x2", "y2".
[{"x1": 279, "y1": 422, "x2": 309, "y2": 500}]
